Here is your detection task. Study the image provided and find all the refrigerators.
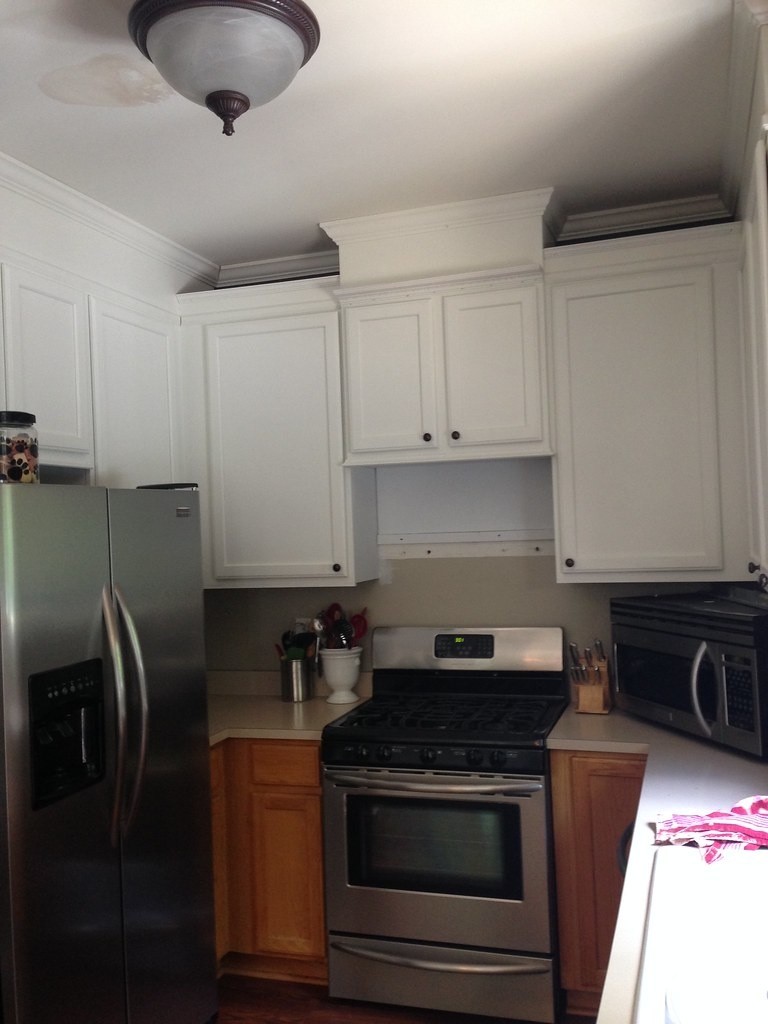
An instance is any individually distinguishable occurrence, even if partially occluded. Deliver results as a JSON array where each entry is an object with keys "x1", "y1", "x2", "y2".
[{"x1": 0, "y1": 486, "x2": 223, "y2": 1024}]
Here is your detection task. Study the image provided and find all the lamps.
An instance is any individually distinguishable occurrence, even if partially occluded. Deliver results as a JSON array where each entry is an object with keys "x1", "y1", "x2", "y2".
[{"x1": 127, "y1": 0, "x2": 322, "y2": 137}]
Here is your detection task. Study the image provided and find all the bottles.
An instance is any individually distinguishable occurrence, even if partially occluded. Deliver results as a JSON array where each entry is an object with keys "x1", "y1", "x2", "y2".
[{"x1": 0, "y1": 411, "x2": 39, "y2": 483}]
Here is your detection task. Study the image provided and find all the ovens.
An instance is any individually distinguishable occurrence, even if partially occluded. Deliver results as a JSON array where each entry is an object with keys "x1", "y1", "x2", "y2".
[{"x1": 321, "y1": 770, "x2": 556, "y2": 1024}]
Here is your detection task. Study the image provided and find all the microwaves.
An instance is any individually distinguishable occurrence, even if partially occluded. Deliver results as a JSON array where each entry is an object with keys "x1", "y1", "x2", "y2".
[{"x1": 609, "y1": 593, "x2": 768, "y2": 759}]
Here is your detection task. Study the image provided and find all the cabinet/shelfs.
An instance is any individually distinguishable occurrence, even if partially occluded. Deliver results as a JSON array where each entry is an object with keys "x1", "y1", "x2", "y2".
[
  {"x1": 88, "y1": 296, "x2": 182, "y2": 487},
  {"x1": 188, "y1": 307, "x2": 381, "y2": 587},
  {"x1": 339, "y1": 276, "x2": 554, "y2": 468},
  {"x1": 550, "y1": 259, "x2": 744, "y2": 583},
  {"x1": 236, "y1": 738, "x2": 325, "y2": 958},
  {"x1": 570, "y1": 751, "x2": 648, "y2": 991},
  {"x1": 736, "y1": 134, "x2": 768, "y2": 592},
  {"x1": 2, "y1": 262, "x2": 93, "y2": 460}
]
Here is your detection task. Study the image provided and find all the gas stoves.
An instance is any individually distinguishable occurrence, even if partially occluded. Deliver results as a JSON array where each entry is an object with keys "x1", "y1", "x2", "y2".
[{"x1": 321, "y1": 627, "x2": 568, "y2": 769}]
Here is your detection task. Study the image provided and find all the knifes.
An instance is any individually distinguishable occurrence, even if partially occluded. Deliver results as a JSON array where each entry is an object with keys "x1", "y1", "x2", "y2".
[{"x1": 569, "y1": 638, "x2": 605, "y2": 685}]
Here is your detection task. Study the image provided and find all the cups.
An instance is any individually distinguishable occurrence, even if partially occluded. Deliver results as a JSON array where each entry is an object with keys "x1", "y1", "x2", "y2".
[
  {"x1": 281, "y1": 660, "x2": 313, "y2": 702},
  {"x1": 320, "y1": 647, "x2": 363, "y2": 703}
]
